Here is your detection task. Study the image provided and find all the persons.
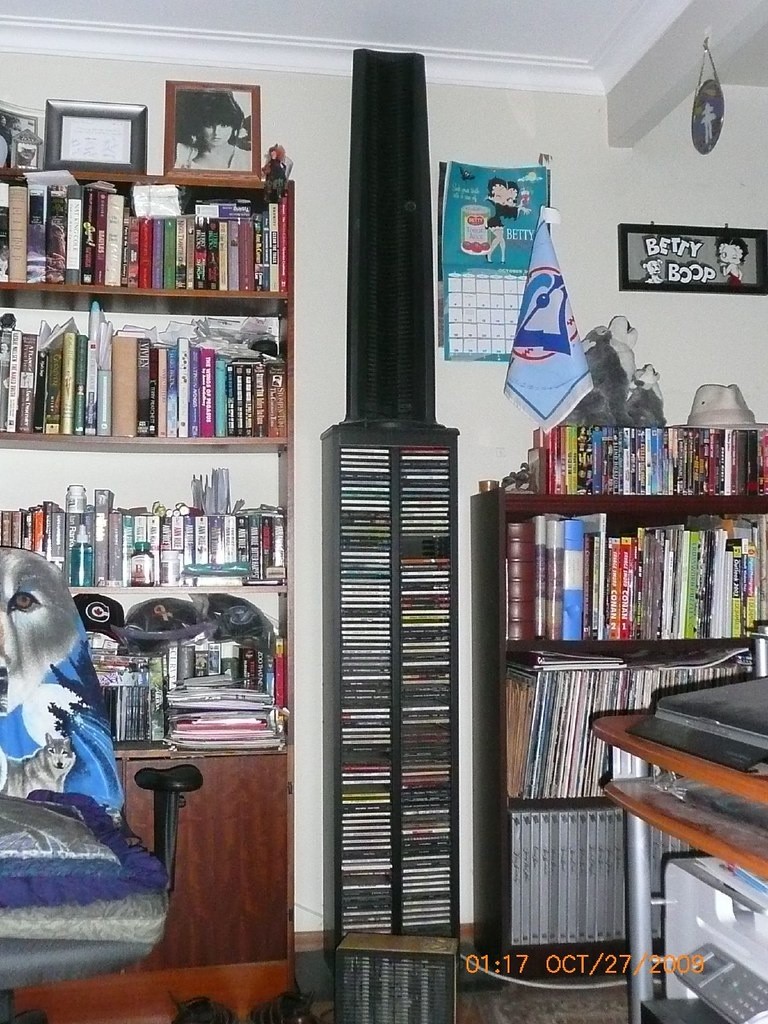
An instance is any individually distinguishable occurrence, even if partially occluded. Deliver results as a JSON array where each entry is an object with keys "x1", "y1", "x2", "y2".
[{"x1": 174, "y1": 90, "x2": 252, "y2": 171}]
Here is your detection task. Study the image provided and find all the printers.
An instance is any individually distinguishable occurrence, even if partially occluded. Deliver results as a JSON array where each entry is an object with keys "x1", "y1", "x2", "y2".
[{"x1": 663, "y1": 856, "x2": 768, "y2": 1024}]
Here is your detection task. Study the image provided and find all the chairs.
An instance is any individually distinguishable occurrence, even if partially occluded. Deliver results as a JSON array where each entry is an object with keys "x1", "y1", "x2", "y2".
[{"x1": 1, "y1": 543, "x2": 205, "y2": 1024}]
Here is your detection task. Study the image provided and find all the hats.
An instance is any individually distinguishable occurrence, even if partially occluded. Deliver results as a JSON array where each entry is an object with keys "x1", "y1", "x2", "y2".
[
  {"x1": 72, "y1": 594, "x2": 276, "y2": 657},
  {"x1": 673, "y1": 384, "x2": 768, "y2": 430}
]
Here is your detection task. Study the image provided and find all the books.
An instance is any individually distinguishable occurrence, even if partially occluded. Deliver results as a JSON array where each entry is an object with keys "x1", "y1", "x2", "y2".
[
  {"x1": 0, "y1": 184, "x2": 288, "y2": 750},
  {"x1": 341, "y1": 426, "x2": 768, "y2": 944}
]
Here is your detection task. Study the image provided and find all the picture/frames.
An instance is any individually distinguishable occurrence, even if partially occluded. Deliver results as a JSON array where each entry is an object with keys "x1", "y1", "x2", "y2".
[
  {"x1": 617, "y1": 222, "x2": 768, "y2": 298},
  {"x1": 164, "y1": 79, "x2": 262, "y2": 180},
  {"x1": 0, "y1": 99, "x2": 44, "y2": 171},
  {"x1": 44, "y1": 99, "x2": 150, "y2": 173}
]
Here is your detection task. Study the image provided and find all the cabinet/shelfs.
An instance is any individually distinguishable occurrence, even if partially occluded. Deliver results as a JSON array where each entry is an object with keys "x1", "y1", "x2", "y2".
[
  {"x1": 1, "y1": 166, "x2": 293, "y2": 966},
  {"x1": 590, "y1": 712, "x2": 768, "y2": 1024},
  {"x1": 467, "y1": 478, "x2": 768, "y2": 979}
]
